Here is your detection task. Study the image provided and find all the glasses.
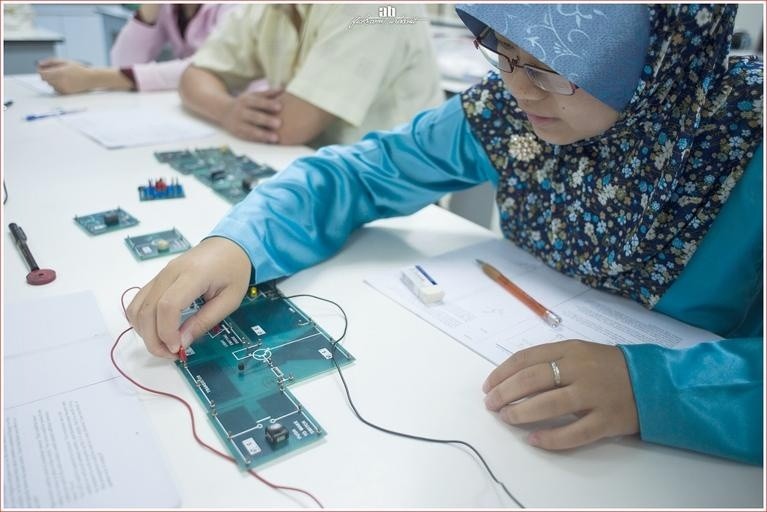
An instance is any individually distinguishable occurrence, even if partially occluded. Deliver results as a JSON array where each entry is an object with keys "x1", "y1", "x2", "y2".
[{"x1": 473, "y1": 24, "x2": 584, "y2": 97}]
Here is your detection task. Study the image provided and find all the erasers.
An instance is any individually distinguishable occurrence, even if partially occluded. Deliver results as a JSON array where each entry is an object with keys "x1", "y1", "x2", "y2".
[{"x1": 401, "y1": 266, "x2": 444, "y2": 304}]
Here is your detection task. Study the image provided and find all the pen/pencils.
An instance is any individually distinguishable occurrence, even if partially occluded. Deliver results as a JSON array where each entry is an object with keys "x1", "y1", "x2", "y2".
[
  {"x1": 476, "y1": 259, "x2": 562, "y2": 328},
  {"x1": 27, "y1": 107, "x2": 87, "y2": 121},
  {"x1": 8, "y1": 223, "x2": 40, "y2": 272}
]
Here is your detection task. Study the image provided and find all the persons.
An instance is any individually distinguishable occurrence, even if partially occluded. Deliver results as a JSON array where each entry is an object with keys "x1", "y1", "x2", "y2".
[
  {"x1": 126, "y1": 3, "x2": 763, "y2": 471},
  {"x1": 178, "y1": 3, "x2": 452, "y2": 212},
  {"x1": 35, "y1": 4, "x2": 236, "y2": 95}
]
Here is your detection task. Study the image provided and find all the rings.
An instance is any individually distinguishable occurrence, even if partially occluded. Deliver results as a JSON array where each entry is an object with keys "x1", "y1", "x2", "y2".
[{"x1": 550, "y1": 361, "x2": 567, "y2": 386}]
